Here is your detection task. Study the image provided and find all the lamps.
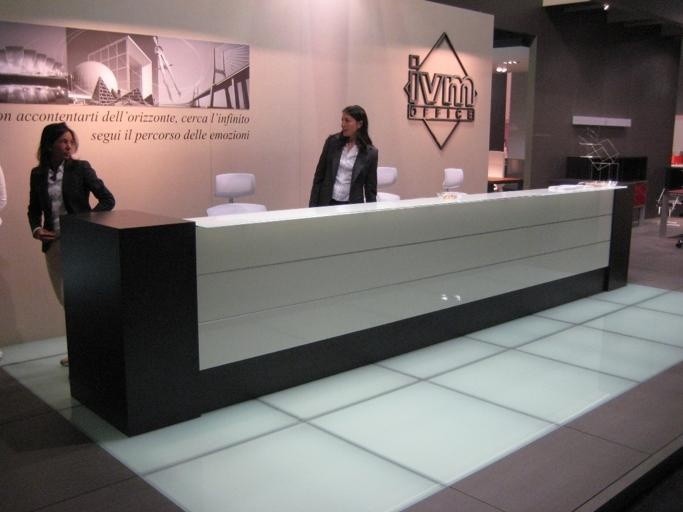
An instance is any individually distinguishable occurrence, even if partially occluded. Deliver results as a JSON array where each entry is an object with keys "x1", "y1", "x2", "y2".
[{"x1": 603, "y1": 2, "x2": 610, "y2": 12}]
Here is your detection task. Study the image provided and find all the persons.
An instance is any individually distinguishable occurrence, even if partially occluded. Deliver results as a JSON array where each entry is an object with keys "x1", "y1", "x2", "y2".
[
  {"x1": 27, "y1": 122, "x2": 114, "y2": 367},
  {"x1": 309, "y1": 105, "x2": 377, "y2": 207}
]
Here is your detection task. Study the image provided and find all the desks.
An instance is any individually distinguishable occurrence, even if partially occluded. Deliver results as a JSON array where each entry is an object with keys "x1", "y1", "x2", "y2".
[{"x1": 488, "y1": 177, "x2": 523, "y2": 193}]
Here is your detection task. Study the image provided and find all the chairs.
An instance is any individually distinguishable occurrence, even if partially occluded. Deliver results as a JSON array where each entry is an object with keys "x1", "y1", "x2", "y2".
[
  {"x1": 442, "y1": 168, "x2": 464, "y2": 192},
  {"x1": 376, "y1": 167, "x2": 400, "y2": 203},
  {"x1": 206, "y1": 174, "x2": 267, "y2": 216}
]
